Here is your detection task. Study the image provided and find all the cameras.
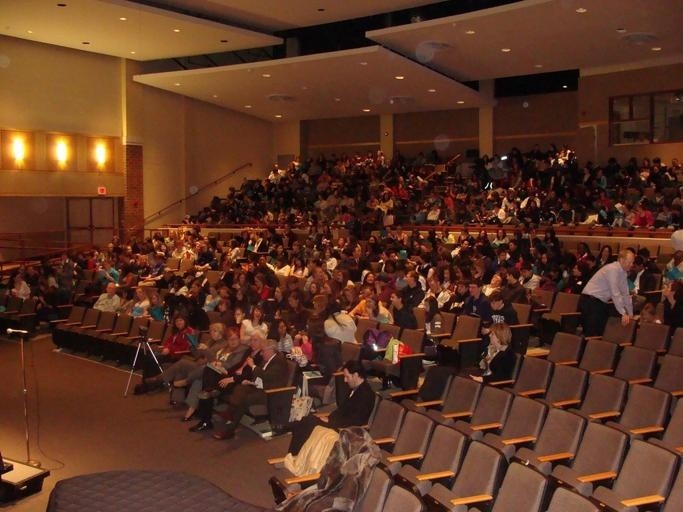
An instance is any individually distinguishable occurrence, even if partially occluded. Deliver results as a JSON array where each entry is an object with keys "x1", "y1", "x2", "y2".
[{"x1": 136, "y1": 325, "x2": 150, "y2": 337}]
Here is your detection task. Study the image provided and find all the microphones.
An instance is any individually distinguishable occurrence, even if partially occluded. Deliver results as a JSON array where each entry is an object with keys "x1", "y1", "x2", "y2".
[{"x1": 5, "y1": 325, "x2": 29, "y2": 333}]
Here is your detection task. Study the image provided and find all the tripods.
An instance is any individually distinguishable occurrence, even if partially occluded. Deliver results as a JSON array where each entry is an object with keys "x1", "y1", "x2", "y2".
[{"x1": 122, "y1": 338, "x2": 172, "y2": 396}]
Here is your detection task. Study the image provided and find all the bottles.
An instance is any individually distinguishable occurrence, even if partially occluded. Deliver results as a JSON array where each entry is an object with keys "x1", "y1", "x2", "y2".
[{"x1": 392, "y1": 344, "x2": 400, "y2": 364}]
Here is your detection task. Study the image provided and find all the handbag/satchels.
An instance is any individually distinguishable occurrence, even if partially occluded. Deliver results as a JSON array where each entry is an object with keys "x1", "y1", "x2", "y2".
[{"x1": 289, "y1": 394, "x2": 313, "y2": 422}]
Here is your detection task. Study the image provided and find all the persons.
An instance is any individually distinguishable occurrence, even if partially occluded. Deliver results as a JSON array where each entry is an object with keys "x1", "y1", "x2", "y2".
[
  {"x1": 639, "y1": 302, "x2": 662, "y2": 324},
  {"x1": 181, "y1": 133, "x2": 683, "y2": 224},
  {"x1": 12, "y1": 228, "x2": 591, "y2": 456},
  {"x1": 596, "y1": 245, "x2": 612, "y2": 270},
  {"x1": 632, "y1": 258, "x2": 657, "y2": 308},
  {"x1": 661, "y1": 281, "x2": 682, "y2": 330},
  {"x1": 576, "y1": 249, "x2": 634, "y2": 333},
  {"x1": 638, "y1": 248, "x2": 658, "y2": 272}
]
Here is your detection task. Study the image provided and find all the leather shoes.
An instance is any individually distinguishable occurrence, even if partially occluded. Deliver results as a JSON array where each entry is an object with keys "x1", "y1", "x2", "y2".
[
  {"x1": 161, "y1": 381, "x2": 176, "y2": 388},
  {"x1": 189, "y1": 422, "x2": 214, "y2": 433},
  {"x1": 180, "y1": 408, "x2": 198, "y2": 422},
  {"x1": 213, "y1": 430, "x2": 236, "y2": 440}
]
